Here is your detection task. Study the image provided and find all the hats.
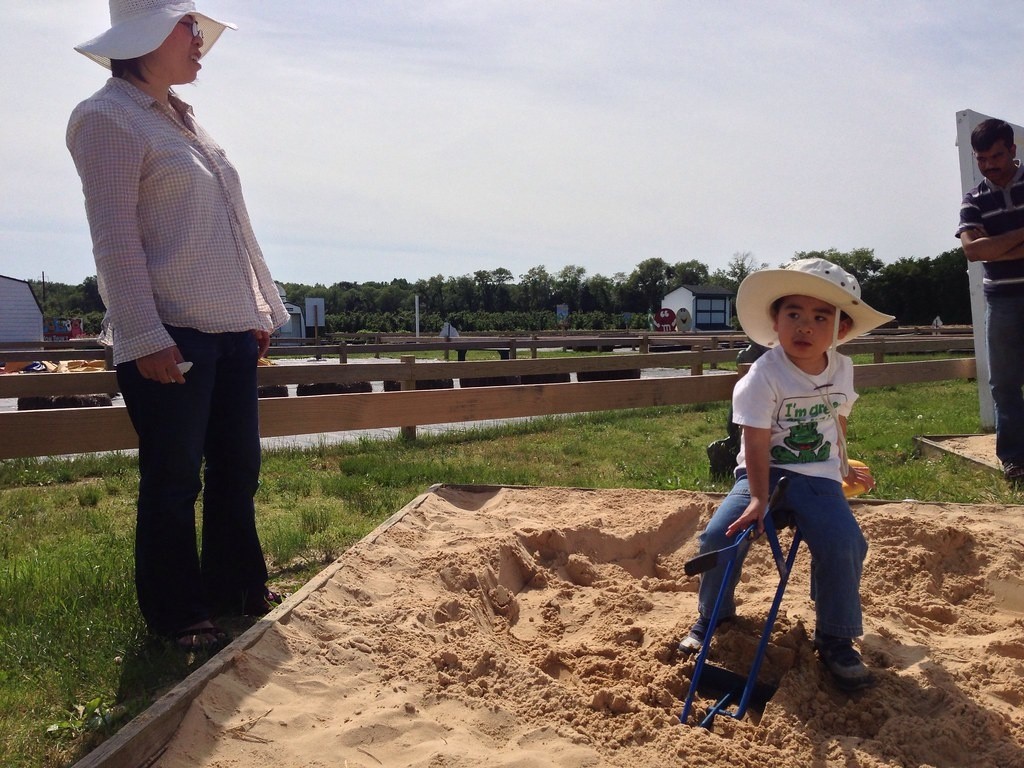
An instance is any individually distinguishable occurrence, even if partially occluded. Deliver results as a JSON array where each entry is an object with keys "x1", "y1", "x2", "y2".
[
  {"x1": 736, "y1": 258, "x2": 895, "y2": 348},
  {"x1": 74, "y1": 0, "x2": 238, "y2": 70}
]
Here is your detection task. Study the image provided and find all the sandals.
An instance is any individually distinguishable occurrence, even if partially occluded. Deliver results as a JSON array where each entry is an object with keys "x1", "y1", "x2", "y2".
[
  {"x1": 172, "y1": 622, "x2": 227, "y2": 652},
  {"x1": 1003, "y1": 458, "x2": 1024, "y2": 478},
  {"x1": 221, "y1": 590, "x2": 290, "y2": 615}
]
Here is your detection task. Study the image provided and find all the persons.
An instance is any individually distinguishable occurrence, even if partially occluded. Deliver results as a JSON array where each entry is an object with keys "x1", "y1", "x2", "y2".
[
  {"x1": 680, "y1": 255, "x2": 896, "y2": 693},
  {"x1": 955, "y1": 119, "x2": 1024, "y2": 487},
  {"x1": 66, "y1": 0, "x2": 285, "y2": 652}
]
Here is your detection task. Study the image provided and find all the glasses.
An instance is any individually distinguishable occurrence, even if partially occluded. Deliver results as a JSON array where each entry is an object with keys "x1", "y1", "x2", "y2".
[{"x1": 179, "y1": 21, "x2": 202, "y2": 38}]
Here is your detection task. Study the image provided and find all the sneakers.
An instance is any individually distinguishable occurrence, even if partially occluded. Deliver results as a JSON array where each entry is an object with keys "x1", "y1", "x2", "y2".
[
  {"x1": 815, "y1": 629, "x2": 877, "y2": 691},
  {"x1": 679, "y1": 614, "x2": 736, "y2": 654}
]
